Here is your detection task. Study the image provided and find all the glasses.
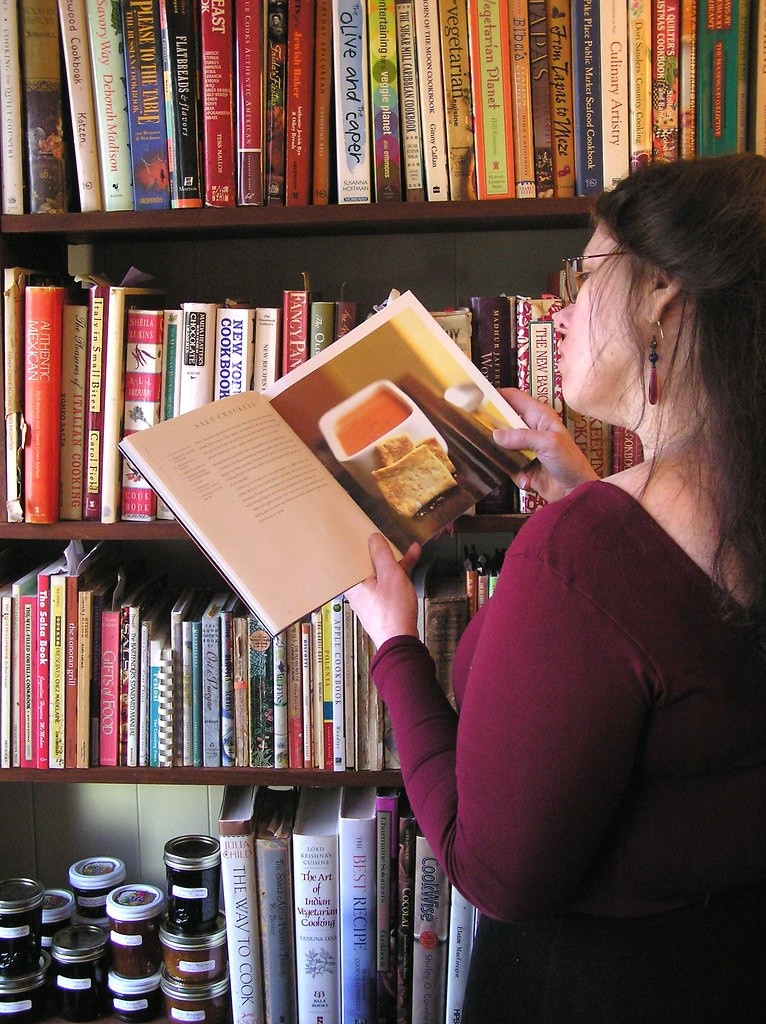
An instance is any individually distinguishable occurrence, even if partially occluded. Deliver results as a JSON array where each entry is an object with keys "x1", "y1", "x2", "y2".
[{"x1": 562, "y1": 251, "x2": 632, "y2": 304}]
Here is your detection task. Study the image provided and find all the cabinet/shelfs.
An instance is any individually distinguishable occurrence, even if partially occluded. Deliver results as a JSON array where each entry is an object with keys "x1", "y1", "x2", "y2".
[{"x1": 0, "y1": 0, "x2": 766, "y2": 1024}]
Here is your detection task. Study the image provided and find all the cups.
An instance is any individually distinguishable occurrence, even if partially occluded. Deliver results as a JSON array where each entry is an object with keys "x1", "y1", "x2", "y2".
[{"x1": 319, "y1": 380, "x2": 448, "y2": 498}]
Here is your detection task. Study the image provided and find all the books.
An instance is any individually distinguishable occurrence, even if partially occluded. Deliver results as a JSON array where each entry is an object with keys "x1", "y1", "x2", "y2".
[
  {"x1": 0, "y1": 0, "x2": 766, "y2": 215},
  {"x1": 0, "y1": 539, "x2": 510, "y2": 772},
  {"x1": 116, "y1": 290, "x2": 540, "y2": 637},
  {"x1": 0, "y1": 243, "x2": 645, "y2": 522}
]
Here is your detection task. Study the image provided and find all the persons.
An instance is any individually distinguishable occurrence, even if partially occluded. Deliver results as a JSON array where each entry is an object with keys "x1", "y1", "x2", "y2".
[
  {"x1": 343, "y1": 154, "x2": 766, "y2": 1024},
  {"x1": 215, "y1": 784, "x2": 480, "y2": 1024}
]
